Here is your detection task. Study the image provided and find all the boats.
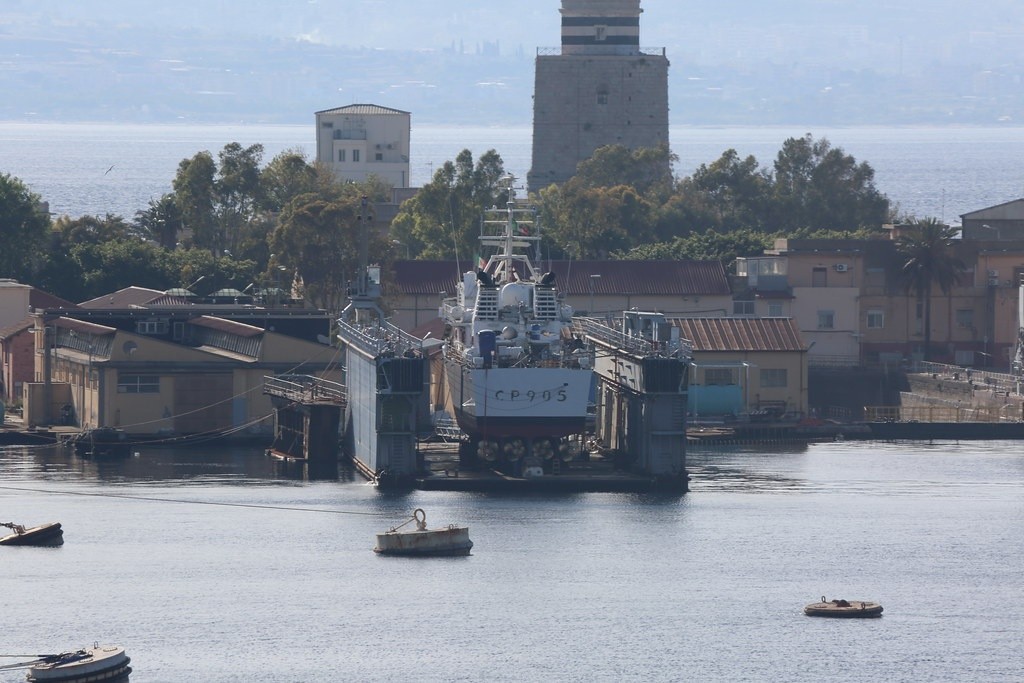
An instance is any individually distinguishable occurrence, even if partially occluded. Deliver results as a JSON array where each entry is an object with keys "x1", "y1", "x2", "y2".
[{"x1": 434, "y1": 167, "x2": 696, "y2": 474}]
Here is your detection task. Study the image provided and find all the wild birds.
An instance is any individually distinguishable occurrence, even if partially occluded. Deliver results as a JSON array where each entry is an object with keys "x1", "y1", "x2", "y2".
[{"x1": 103, "y1": 164, "x2": 116, "y2": 176}]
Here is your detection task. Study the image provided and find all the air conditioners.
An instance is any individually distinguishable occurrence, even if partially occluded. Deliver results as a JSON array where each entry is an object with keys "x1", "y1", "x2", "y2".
[
  {"x1": 836, "y1": 263, "x2": 848, "y2": 271},
  {"x1": 988, "y1": 270, "x2": 999, "y2": 277},
  {"x1": 988, "y1": 279, "x2": 999, "y2": 285}
]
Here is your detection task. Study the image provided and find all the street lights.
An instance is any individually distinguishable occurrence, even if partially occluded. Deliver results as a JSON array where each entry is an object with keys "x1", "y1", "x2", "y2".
[
  {"x1": 692, "y1": 362, "x2": 700, "y2": 429},
  {"x1": 742, "y1": 361, "x2": 751, "y2": 417}
]
